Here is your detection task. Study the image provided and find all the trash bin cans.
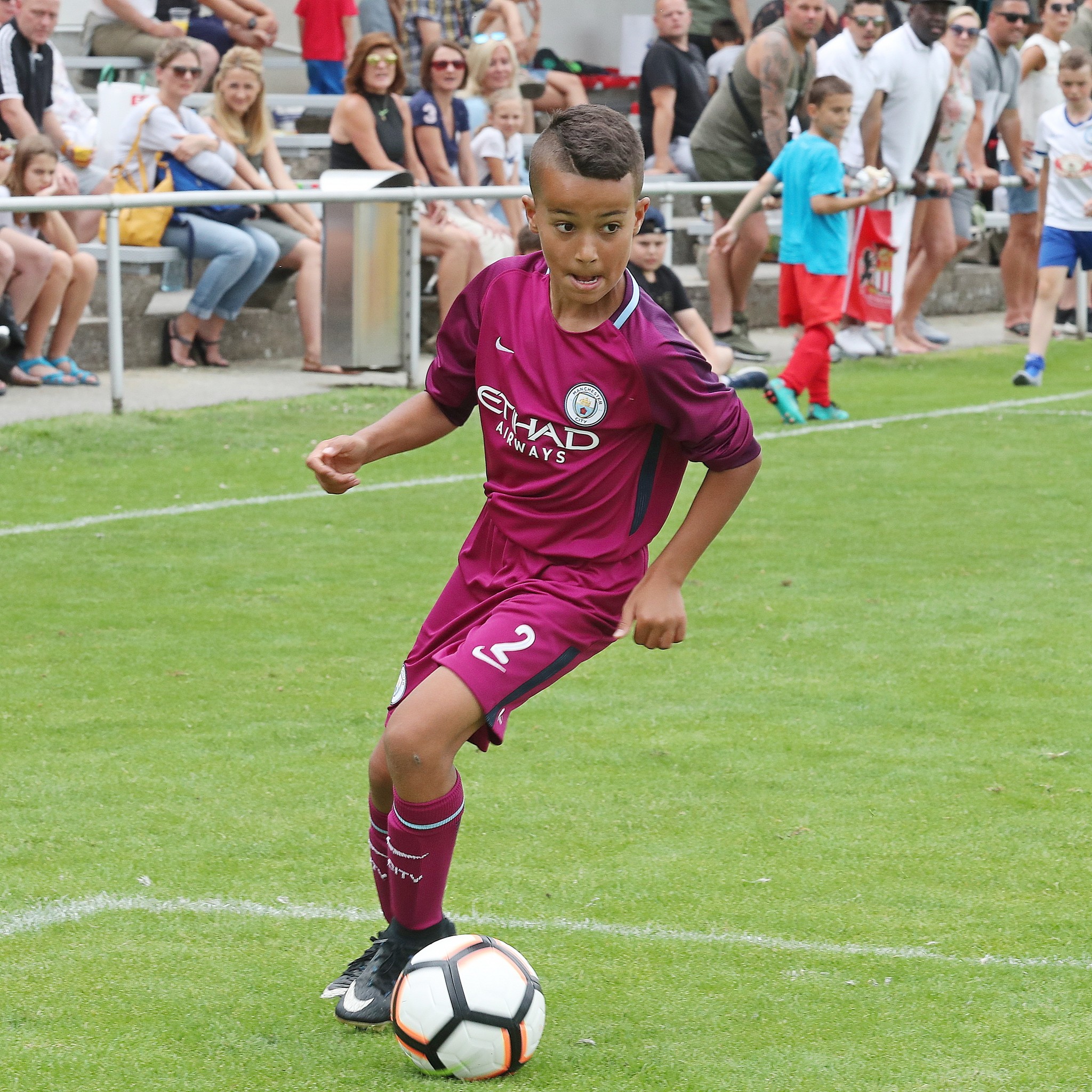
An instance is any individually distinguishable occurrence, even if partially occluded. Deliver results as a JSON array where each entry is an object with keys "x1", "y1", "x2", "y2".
[{"x1": 316, "y1": 167, "x2": 416, "y2": 371}]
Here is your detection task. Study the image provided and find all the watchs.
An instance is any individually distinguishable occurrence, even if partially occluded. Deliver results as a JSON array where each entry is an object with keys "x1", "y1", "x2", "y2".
[{"x1": 247, "y1": 17, "x2": 257, "y2": 28}]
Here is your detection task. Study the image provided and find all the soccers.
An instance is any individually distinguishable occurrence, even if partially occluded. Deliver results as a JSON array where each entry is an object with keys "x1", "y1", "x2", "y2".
[{"x1": 389, "y1": 933, "x2": 546, "y2": 1082}]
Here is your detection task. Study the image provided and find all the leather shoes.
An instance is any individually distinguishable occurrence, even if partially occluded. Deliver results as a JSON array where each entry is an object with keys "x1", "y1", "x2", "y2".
[{"x1": 10, "y1": 366, "x2": 43, "y2": 387}]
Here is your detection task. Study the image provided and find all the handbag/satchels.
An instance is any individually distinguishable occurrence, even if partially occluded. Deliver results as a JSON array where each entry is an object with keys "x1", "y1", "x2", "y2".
[
  {"x1": 99, "y1": 169, "x2": 174, "y2": 246},
  {"x1": 753, "y1": 131, "x2": 792, "y2": 196}
]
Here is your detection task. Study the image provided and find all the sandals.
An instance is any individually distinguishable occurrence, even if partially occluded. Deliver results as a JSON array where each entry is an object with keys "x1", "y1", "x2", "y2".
[
  {"x1": 164, "y1": 317, "x2": 196, "y2": 369},
  {"x1": 303, "y1": 357, "x2": 340, "y2": 374},
  {"x1": 195, "y1": 334, "x2": 229, "y2": 368},
  {"x1": 18, "y1": 357, "x2": 79, "y2": 385},
  {"x1": 51, "y1": 357, "x2": 101, "y2": 386}
]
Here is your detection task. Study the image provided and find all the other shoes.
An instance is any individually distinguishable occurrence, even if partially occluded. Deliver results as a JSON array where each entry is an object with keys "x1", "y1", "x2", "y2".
[
  {"x1": 714, "y1": 305, "x2": 949, "y2": 362},
  {"x1": 1006, "y1": 300, "x2": 1092, "y2": 339}
]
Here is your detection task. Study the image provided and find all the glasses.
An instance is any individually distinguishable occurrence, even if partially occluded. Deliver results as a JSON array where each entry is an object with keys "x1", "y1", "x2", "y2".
[
  {"x1": 849, "y1": 15, "x2": 885, "y2": 27},
  {"x1": 430, "y1": 58, "x2": 464, "y2": 70},
  {"x1": 165, "y1": 65, "x2": 203, "y2": 78},
  {"x1": 996, "y1": 12, "x2": 1031, "y2": 23},
  {"x1": 1050, "y1": 3, "x2": 1075, "y2": 12},
  {"x1": 473, "y1": 31, "x2": 505, "y2": 44},
  {"x1": 366, "y1": 53, "x2": 397, "y2": 66},
  {"x1": 950, "y1": 24, "x2": 979, "y2": 39}
]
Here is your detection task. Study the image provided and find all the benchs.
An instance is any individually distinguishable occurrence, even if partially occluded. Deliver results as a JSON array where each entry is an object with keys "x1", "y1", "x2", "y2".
[{"x1": 62, "y1": 59, "x2": 692, "y2": 375}]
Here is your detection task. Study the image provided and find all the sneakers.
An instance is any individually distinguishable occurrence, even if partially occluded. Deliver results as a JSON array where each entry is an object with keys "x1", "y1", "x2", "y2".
[
  {"x1": 808, "y1": 401, "x2": 848, "y2": 421},
  {"x1": 764, "y1": 378, "x2": 806, "y2": 425},
  {"x1": 320, "y1": 930, "x2": 389, "y2": 999},
  {"x1": 334, "y1": 914, "x2": 456, "y2": 1026},
  {"x1": 1014, "y1": 356, "x2": 1045, "y2": 386},
  {"x1": 729, "y1": 366, "x2": 770, "y2": 387}
]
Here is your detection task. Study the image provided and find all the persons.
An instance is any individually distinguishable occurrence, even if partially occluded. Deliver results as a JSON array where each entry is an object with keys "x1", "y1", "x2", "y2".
[
  {"x1": 304, "y1": 104, "x2": 761, "y2": 1024},
  {"x1": 0, "y1": 0, "x2": 1092, "y2": 424}
]
